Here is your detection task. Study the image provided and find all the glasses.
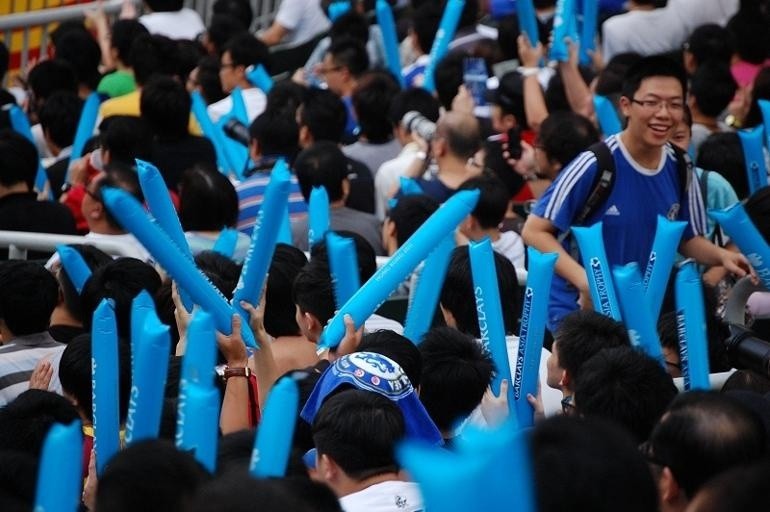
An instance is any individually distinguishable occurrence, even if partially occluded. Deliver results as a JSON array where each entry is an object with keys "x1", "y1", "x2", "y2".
[
  {"x1": 560, "y1": 395, "x2": 578, "y2": 417},
  {"x1": 631, "y1": 96, "x2": 688, "y2": 114}
]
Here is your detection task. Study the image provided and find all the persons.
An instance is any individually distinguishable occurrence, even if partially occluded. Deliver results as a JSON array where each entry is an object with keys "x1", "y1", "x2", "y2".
[{"x1": 1, "y1": 1, "x2": 770, "y2": 511}]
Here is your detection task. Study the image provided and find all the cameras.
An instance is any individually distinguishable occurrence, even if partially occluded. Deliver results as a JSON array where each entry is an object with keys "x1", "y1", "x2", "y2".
[
  {"x1": 400, "y1": 109, "x2": 437, "y2": 141},
  {"x1": 223, "y1": 116, "x2": 252, "y2": 148},
  {"x1": 722, "y1": 323, "x2": 770, "y2": 376}
]
